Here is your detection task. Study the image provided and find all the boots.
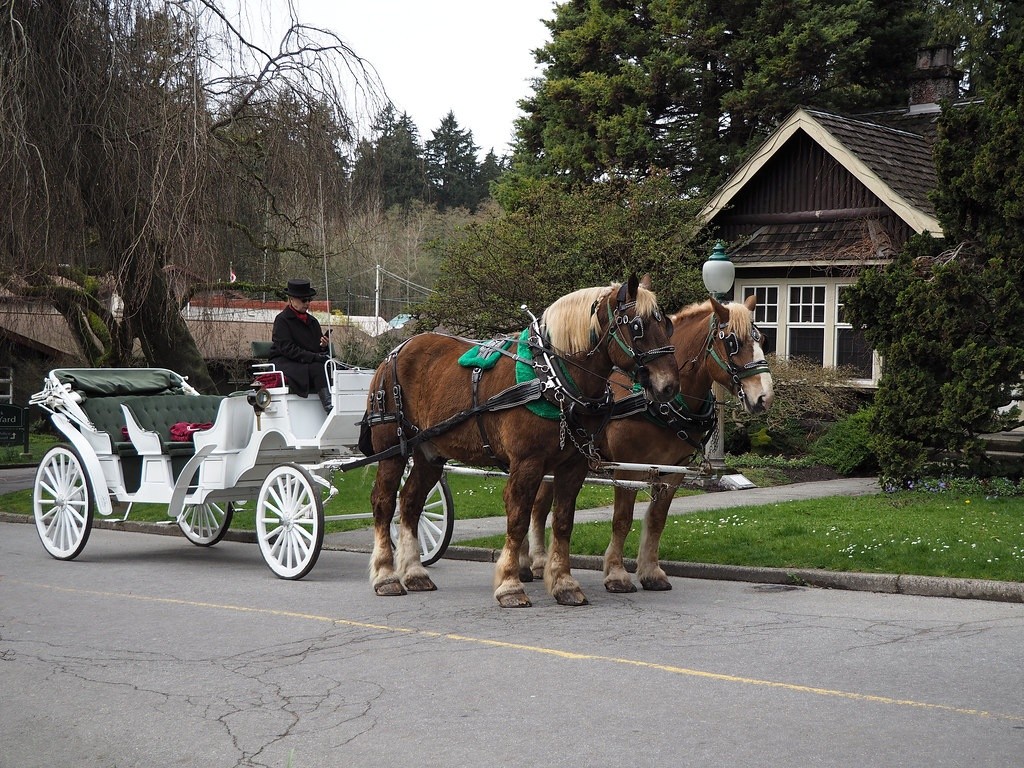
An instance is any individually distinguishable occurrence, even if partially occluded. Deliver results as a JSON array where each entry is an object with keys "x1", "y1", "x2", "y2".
[{"x1": 318, "y1": 387, "x2": 334, "y2": 415}]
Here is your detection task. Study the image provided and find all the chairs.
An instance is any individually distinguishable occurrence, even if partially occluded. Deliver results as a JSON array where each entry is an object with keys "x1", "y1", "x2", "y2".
[{"x1": 250, "y1": 341, "x2": 331, "y2": 436}]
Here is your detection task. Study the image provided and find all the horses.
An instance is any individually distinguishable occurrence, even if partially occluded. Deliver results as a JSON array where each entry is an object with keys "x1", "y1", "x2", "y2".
[
  {"x1": 357, "y1": 271, "x2": 682, "y2": 607},
  {"x1": 518, "y1": 295, "x2": 774, "y2": 593}
]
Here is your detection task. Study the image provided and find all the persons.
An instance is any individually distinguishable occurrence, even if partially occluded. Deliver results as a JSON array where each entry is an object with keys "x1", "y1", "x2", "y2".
[{"x1": 268, "y1": 280, "x2": 345, "y2": 416}]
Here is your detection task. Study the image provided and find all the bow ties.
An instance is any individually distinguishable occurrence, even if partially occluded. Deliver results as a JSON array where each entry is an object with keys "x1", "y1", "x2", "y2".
[{"x1": 296, "y1": 313, "x2": 308, "y2": 319}]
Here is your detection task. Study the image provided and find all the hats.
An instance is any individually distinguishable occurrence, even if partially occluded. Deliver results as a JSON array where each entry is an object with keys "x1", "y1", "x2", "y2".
[{"x1": 283, "y1": 279, "x2": 317, "y2": 297}]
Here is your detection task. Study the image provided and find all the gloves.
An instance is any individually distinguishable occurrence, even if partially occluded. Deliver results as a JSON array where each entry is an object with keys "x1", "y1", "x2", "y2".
[{"x1": 316, "y1": 351, "x2": 329, "y2": 362}]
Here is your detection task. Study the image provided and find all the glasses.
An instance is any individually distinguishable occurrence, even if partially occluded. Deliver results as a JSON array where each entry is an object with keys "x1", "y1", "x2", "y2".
[{"x1": 297, "y1": 296, "x2": 313, "y2": 304}]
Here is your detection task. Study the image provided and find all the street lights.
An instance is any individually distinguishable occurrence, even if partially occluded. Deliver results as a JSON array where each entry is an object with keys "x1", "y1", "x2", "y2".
[{"x1": 701, "y1": 240, "x2": 742, "y2": 479}]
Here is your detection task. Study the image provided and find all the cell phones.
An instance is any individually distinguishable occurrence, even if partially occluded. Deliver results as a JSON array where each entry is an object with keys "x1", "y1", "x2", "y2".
[{"x1": 323, "y1": 328, "x2": 333, "y2": 339}]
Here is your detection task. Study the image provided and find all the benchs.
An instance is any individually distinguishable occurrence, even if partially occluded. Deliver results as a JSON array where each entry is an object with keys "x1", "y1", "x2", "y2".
[{"x1": 80, "y1": 395, "x2": 231, "y2": 493}]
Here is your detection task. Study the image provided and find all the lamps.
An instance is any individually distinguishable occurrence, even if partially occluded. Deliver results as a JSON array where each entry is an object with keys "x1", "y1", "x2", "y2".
[{"x1": 703, "y1": 240, "x2": 735, "y2": 299}]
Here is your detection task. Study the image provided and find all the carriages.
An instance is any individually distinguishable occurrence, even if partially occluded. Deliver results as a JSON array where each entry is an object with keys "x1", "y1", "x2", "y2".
[{"x1": 27, "y1": 266, "x2": 777, "y2": 608}]
